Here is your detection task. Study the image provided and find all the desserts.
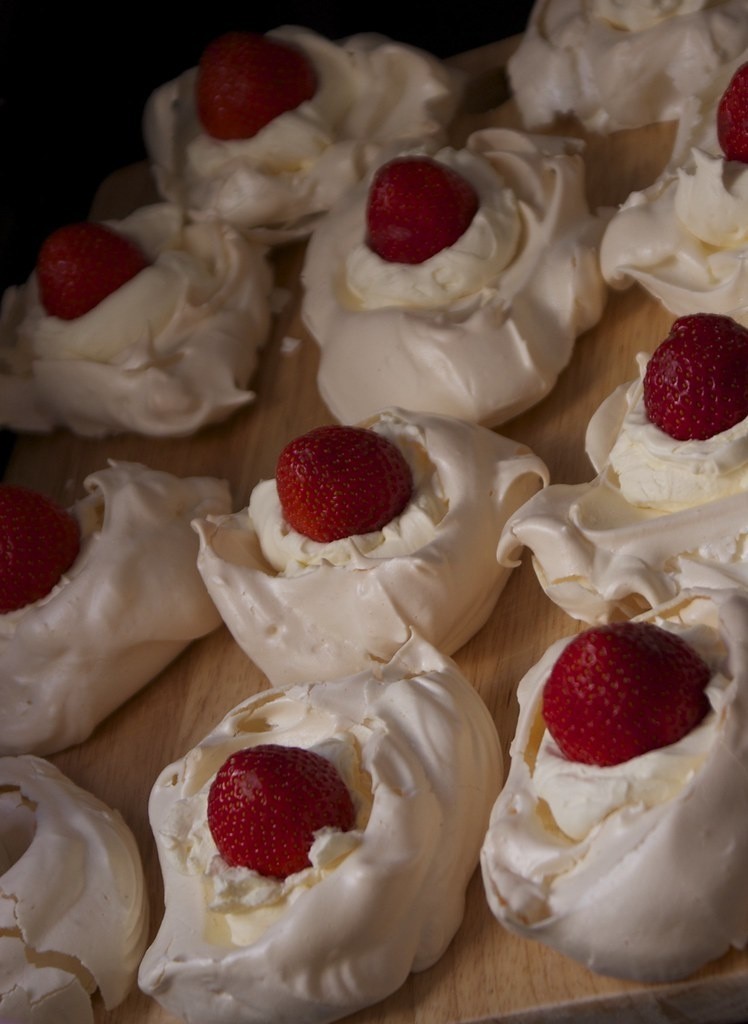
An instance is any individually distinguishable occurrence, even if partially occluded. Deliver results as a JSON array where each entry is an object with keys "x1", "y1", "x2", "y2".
[{"x1": 0, "y1": 1, "x2": 748, "y2": 1024}]
